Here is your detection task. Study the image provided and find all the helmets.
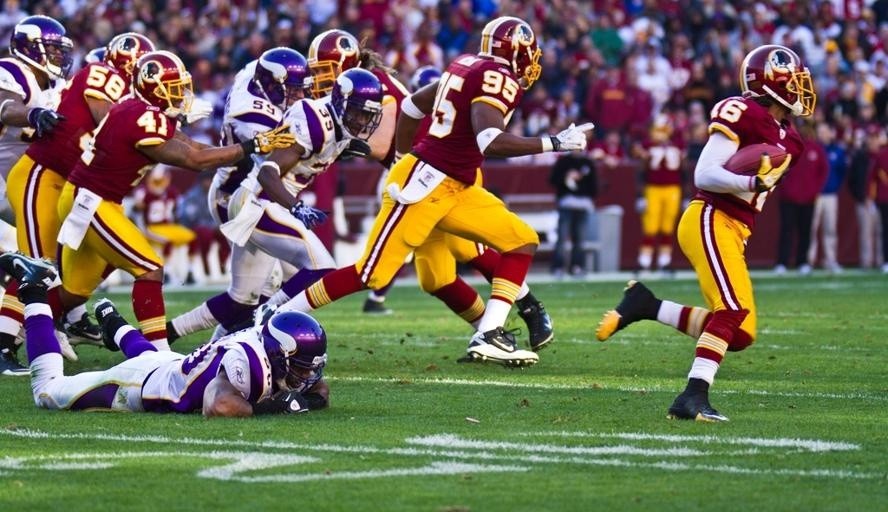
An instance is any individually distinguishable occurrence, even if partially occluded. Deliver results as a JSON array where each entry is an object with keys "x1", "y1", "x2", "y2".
[
  {"x1": 10, "y1": 15, "x2": 65, "y2": 80},
  {"x1": 307, "y1": 29, "x2": 362, "y2": 100},
  {"x1": 103, "y1": 31, "x2": 154, "y2": 84},
  {"x1": 410, "y1": 65, "x2": 441, "y2": 90},
  {"x1": 61, "y1": 36, "x2": 73, "y2": 76},
  {"x1": 739, "y1": 44, "x2": 817, "y2": 119},
  {"x1": 261, "y1": 310, "x2": 327, "y2": 394},
  {"x1": 477, "y1": 16, "x2": 542, "y2": 91},
  {"x1": 253, "y1": 47, "x2": 313, "y2": 113},
  {"x1": 131, "y1": 50, "x2": 194, "y2": 119},
  {"x1": 330, "y1": 67, "x2": 383, "y2": 143},
  {"x1": 84, "y1": 46, "x2": 108, "y2": 63}
]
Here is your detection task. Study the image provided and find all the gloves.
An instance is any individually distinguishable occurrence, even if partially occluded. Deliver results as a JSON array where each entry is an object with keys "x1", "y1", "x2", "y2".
[
  {"x1": 26, "y1": 107, "x2": 65, "y2": 138},
  {"x1": 250, "y1": 393, "x2": 327, "y2": 417},
  {"x1": 345, "y1": 139, "x2": 371, "y2": 157},
  {"x1": 541, "y1": 122, "x2": 595, "y2": 153},
  {"x1": 240, "y1": 125, "x2": 297, "y2": 156},
  {"x1": 290, "y1": 200, "x2": 331, "y2": 230},
  {"x1": 748, "y1": 152, "x2": 792, "y2": 192}
]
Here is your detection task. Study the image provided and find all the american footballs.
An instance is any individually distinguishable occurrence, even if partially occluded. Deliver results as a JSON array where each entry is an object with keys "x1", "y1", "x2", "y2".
[{"x1": 723, "y1": 143, "x2": 787, "y2": 175}]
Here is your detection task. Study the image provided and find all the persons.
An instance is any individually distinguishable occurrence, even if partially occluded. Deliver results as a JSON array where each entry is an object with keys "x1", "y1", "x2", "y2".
[
  {"x1": 596, "y1": 43, "x2": 816, "y2": 423},
  {"x1": 1, "y1": 251, "x2": 327, "y2": 417},
  {"x1": 0, "y1": 2, "x2": 888, "y2": 365}
]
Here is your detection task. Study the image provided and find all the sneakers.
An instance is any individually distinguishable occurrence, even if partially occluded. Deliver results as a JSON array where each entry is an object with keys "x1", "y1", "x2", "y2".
[
  {"x1": 665, "y1": 390, "x2": 729, "y2": 424},
  {"x1": 53, "y1": 327, "x2": 78, "y2": 364},
  {"x1": 458, "y1": 332, "x2": 512, "y2": 361},
  {"x1": 467, "y1": 326, "x2": 539, "y2": 367},
  {"x1": 596, "y1": 280, "x2": 654, "y2": 342},
  {"x1": 60, "y1": 312, "x2": 107, "y2": 345},
  {"x1": 517, "y1": 301, "x2": 554, "y2": 352},
  {"x1": 363, "y1": 299, "x2": 393, "y2": 317},
  {"x1": 0, "y1": 348, "x2": 32, "y2": 377},
  {"x1": 0, "y1": 251, "x2": 59, "y2": 305},
  {"x1": 93, "y1": 297, "x2": 119, "y2": 353}
]
